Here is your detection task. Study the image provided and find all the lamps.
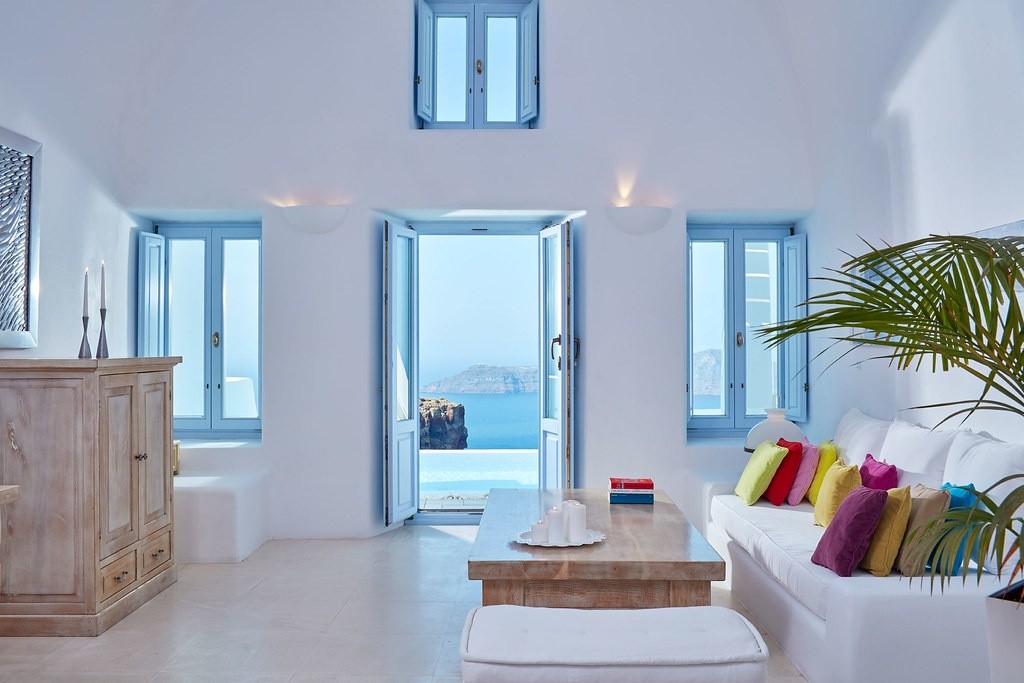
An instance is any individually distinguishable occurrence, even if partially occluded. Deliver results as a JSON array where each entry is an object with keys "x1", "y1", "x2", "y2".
[{"x1": 742, "y1": 408, "x2": 804, "y2": 452}]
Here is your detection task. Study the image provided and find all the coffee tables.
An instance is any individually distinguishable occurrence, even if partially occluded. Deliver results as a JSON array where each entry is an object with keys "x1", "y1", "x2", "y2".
[{"x1": 467, "y1": 487, "x2": 726, "y2": 609}]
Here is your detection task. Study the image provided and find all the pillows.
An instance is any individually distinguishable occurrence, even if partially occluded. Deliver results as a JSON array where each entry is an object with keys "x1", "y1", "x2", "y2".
[{"x1": 733, "y1": 437, "x2": 975, "y2": 575}]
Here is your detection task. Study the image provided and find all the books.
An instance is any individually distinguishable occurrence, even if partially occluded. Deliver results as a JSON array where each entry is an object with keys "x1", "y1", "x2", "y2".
[{"x1": 608, "y1": 478, "x2": 654, "y2": 504}]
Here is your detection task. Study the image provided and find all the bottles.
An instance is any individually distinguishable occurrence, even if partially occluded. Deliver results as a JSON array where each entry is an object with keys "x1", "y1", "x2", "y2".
[
  {"x1": 562, "y1": 499, "x2": 580, "y2": 539},
  {"x1": 531, "y1": 520, "x2": 548, "y2": 545},
  {"x1": 568, "y1": 502, "x2": 586, "y2": 544},
  {"x1": 547, "y1": 507, "x2": 562, "y2": 545}
]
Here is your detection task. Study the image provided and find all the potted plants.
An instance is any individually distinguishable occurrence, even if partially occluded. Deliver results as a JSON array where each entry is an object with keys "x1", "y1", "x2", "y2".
[{"x1": 745, "y1": 233, "x2": 1024, "y2": 681}]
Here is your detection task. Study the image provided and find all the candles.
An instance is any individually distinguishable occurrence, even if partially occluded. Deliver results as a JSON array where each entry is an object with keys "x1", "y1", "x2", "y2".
[
  {"x1": 99, "y1": 259, "x2": 105, "y2": 309},
  {"x1": 83, "y1": 267, "x2": 88, "y2": 317}
]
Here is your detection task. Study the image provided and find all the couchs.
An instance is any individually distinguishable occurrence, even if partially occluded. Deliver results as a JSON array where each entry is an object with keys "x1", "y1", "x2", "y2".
[{"x1": 706, "y1": 408, "x2": 1024, "y2": 682}]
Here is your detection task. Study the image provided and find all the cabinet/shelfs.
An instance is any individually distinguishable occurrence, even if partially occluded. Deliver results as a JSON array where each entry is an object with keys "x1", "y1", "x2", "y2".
[{"x1": 0, "y1": 356, "x2": 183, "y2": 637}]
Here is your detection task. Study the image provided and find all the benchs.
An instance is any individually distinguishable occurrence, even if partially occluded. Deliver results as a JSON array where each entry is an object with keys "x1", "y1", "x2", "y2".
[{"x1": 459, "y1": 604, "x2": 769, "y2": 683}]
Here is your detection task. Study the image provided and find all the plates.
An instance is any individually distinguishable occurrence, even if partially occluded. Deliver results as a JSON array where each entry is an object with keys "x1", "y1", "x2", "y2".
[{"x1": 510, "y1": 527, "x2": 606, "y2": 547}]
